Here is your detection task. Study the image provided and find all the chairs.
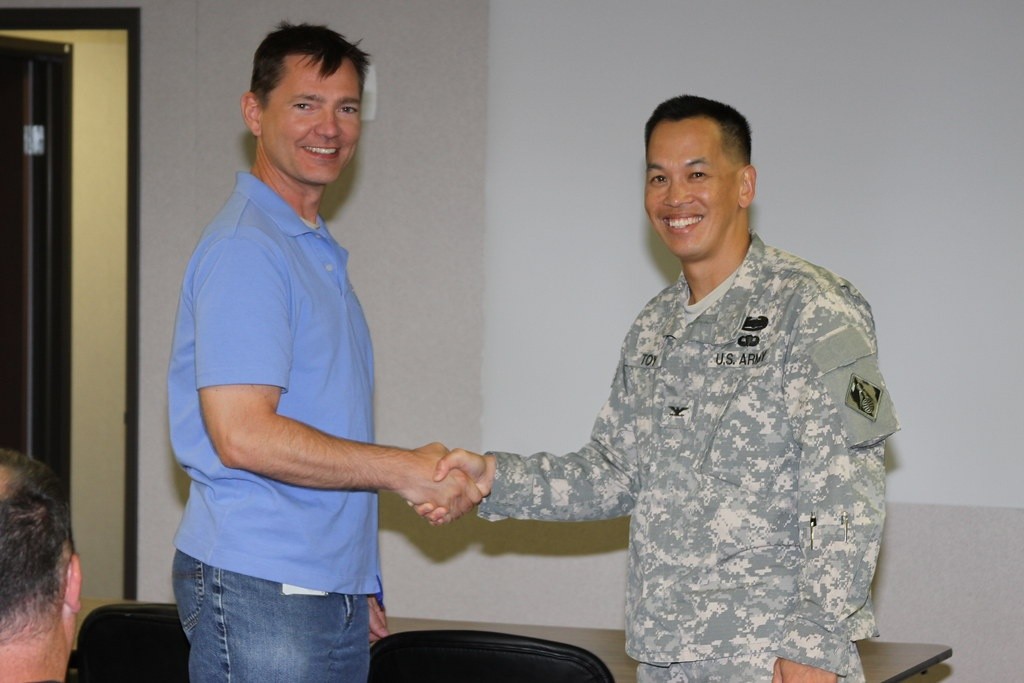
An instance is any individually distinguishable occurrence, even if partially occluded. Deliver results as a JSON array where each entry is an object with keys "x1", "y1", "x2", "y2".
[
  {"x1": 366, "y1": 630, "x2": 616, "y2": 682},
  {"x1": 67, "y1": 601, "x2": 191, "y2": 682}
]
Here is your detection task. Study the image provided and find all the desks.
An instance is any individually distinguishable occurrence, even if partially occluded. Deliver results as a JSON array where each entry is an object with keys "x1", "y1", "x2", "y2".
[{"x1": 73, "y1": 597, "x2": 952, "y2": 682}]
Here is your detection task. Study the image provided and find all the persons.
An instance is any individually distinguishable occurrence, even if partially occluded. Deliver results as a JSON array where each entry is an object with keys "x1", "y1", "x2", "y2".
[
  {"x1": 406, "y1": 93, "x2": 902, "y2": 683},
  {"x1": 168, "y1": 19, "x2": 483, "y2": 683},
  {"x1": 0, "y1": 449, "x2": 84, "y2": 683}
]
